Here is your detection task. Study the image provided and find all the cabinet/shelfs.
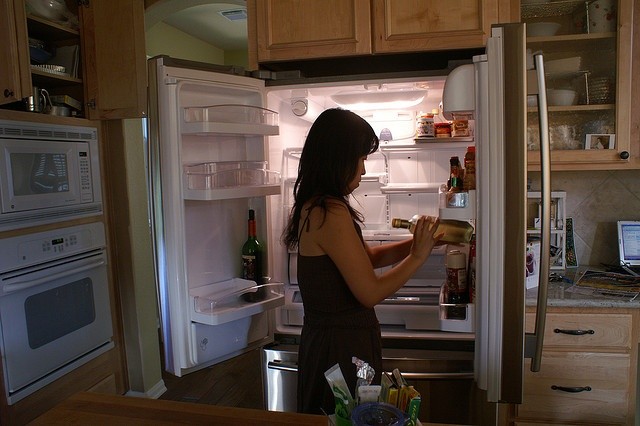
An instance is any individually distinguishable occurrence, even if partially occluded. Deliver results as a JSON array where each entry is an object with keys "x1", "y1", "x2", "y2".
[
  {"x1": 510, "y1": 2, "x2": 640, "y2": 171},
  {"x1": 498, "y1": 306, "x2": 636, "y2": 424},
  {"x1": 2, "y1": 1, "x2": 149, "y2": 123},
  {"x1": 245, "y1": 1, "x2": 497, "y2": 71}
]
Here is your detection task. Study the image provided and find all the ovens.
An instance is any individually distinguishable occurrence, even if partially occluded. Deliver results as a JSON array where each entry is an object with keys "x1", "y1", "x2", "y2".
[{"x1": 0, "y1": 220, "x2": 117, "y2": 405}]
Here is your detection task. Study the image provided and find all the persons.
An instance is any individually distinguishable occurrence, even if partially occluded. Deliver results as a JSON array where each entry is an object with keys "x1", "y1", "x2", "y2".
[
  {"x1": 282, "y1": 108, "x2": 467, "y2": 415},
  {"x1": 591, "y1": 138, "x2": 604, "y2": 150}
]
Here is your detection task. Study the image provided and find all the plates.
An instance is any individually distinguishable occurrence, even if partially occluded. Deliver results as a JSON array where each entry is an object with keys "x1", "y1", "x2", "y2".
[{"x1": 589, "y1": 75, "x2": 615, "y2": 105}]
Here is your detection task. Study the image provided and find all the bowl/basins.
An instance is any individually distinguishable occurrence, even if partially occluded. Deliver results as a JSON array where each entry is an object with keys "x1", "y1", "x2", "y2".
[{"x1": 548, "y1": 89, "x2": 580, "y2": 106}]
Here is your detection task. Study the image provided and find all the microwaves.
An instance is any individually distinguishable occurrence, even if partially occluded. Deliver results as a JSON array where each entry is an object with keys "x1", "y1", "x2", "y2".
[{"x1": 0, "y1": 118, "x2": 104, "y2": 234}]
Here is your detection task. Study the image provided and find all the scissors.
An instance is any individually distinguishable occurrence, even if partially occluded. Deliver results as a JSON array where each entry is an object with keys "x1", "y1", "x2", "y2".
[{"x1": 549, "y1": 272, "x2": 574, "y2": 284}]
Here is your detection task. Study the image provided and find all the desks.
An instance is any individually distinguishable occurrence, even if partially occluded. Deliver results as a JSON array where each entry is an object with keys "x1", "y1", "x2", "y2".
[{"x1": 24, "y1": 390, "x2": 428, "y2": 425}]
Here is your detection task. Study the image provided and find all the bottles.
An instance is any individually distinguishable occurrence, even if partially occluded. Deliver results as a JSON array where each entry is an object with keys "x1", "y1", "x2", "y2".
[
  {"x1": 462, "y1": 145, "x2": 476, "y2": 193},
  {"x1": 447, "y1": 155, "x2": 462, "y2": 192},
  {"x1": 240, "y1": 207, "x2": 264, "y2": 304},
  {"x1": 466, "y1": 233, "x2": 476, "y2": 304},
  {"x1": 448, "y1": 165, "x2": 462, "y2": 207},
  {"x1": 445, "y1": 249, "x2": 468, "y2": 320},
  {"x1": 391, "y1": 214, "x2": 474, "y2": 243}
]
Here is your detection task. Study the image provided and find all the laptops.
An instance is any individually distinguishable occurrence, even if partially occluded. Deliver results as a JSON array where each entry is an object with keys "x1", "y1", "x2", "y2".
[{"x1": 617, "y1": 220, "x2": 640, "y2": 276}]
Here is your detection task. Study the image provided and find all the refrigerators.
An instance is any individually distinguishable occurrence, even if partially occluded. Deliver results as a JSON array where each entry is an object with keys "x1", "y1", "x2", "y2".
[{"x1": 144, "y1": 22, "x2": 553, "y2": 426}]
